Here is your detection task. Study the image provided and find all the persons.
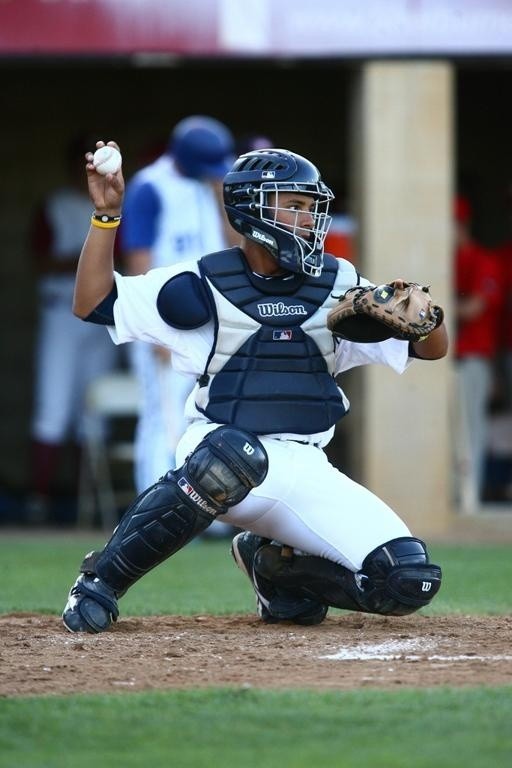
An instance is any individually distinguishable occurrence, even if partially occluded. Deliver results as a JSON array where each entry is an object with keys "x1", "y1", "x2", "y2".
[
  {"x1": 25, "y1": 163, "x2": 122, "y2": 529},
  {"x1": 115, "y1": 116, "x2": 238, "y2": 541},
  {"x1": 455, "y1": 169, "x2": 507, "y2": 523},
  {"x1": 61, "y1": 140, "x2": 448, "y2": 633}
]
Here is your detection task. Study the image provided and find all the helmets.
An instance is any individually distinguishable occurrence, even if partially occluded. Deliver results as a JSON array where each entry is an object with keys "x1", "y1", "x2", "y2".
[
  {"x1": 223, "y1": 148, "x2": 337, "y2": 281},
  {"x1": 168, "y1": 114, "x2": 235, "y2": 179}
]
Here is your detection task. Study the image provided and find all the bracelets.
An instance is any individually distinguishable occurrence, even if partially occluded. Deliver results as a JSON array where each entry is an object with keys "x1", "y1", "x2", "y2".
[{"x1": 90, "y1": 216, "x2": 121, "y2": 228}]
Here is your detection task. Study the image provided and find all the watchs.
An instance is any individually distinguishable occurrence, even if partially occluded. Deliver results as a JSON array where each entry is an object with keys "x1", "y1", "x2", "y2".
[{"x1": 92, "y1": 211, "x2": 123, "y2": 222}]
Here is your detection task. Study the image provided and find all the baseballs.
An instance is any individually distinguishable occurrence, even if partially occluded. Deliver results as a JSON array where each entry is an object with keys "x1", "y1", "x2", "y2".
[{"x1": 92, "y1": 145, "x2": 121, "y2": 175}]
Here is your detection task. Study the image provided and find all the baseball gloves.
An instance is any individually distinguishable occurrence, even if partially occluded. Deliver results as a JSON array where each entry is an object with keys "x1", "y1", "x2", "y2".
[{"x1": 326, "y1": 281, "x2": 442, "y2": 342}]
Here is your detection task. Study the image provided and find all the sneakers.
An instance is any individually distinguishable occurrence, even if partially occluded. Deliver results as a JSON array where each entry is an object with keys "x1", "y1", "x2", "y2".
[
  {"x1": 62, "y1": 572, "x2": 111, "y2": 635},
  {"x1": 230, "y1": 530, "x2": 281, "y2": 624}
]
